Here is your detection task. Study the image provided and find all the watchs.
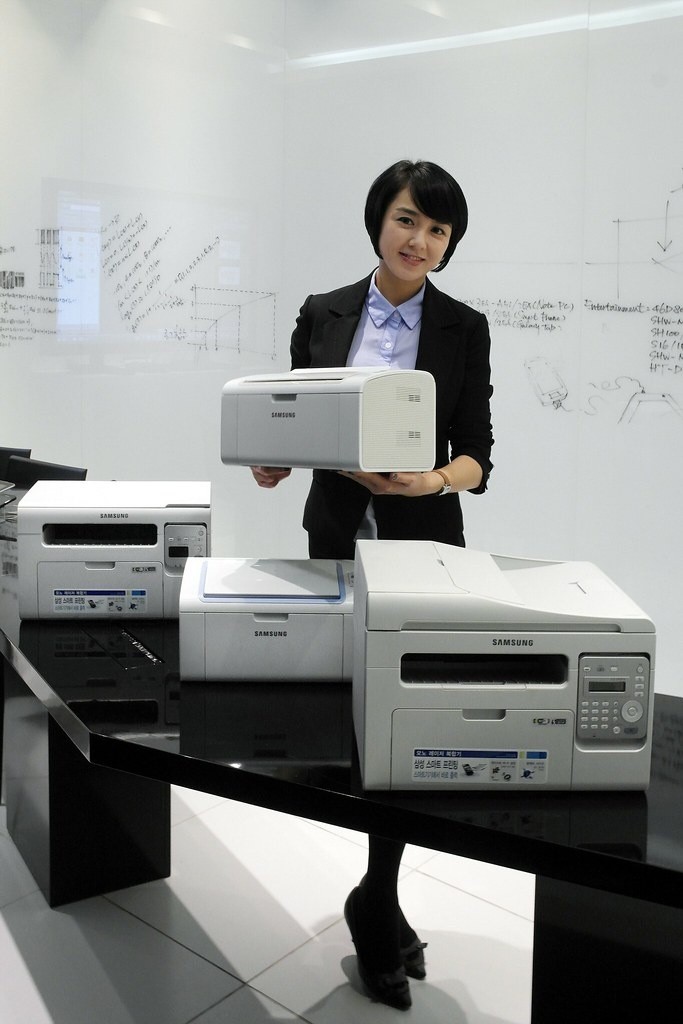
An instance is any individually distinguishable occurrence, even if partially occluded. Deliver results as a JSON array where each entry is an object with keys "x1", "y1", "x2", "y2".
[{"x1": 432, "y1": 470, "x2": 451, "y2": 497}]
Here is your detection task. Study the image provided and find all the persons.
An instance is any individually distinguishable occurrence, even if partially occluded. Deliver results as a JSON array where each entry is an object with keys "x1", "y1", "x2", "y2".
[{"x1": 249, "y1": 160, "x2": 495, "y2": 1006}]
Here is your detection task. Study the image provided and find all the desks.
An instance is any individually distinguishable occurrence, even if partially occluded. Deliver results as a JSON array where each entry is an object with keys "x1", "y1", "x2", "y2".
[{"x1": 0, "y1": 485, "x2": 683, "y2": 1024}]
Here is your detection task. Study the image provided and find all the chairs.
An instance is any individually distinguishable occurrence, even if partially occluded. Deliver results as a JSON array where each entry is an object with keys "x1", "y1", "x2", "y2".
[{"x1": 0, "y1": 446, "x2": 88, "y2": 487}]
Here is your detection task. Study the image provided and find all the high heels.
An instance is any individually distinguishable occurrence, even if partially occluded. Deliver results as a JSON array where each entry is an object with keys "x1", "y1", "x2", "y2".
[
  {"x1": 359, "y1": 873, "x2": 429, "y2": 980},
  {"x1": 344, "y1": 886, "x2": 412, "y2": 1010}
]
